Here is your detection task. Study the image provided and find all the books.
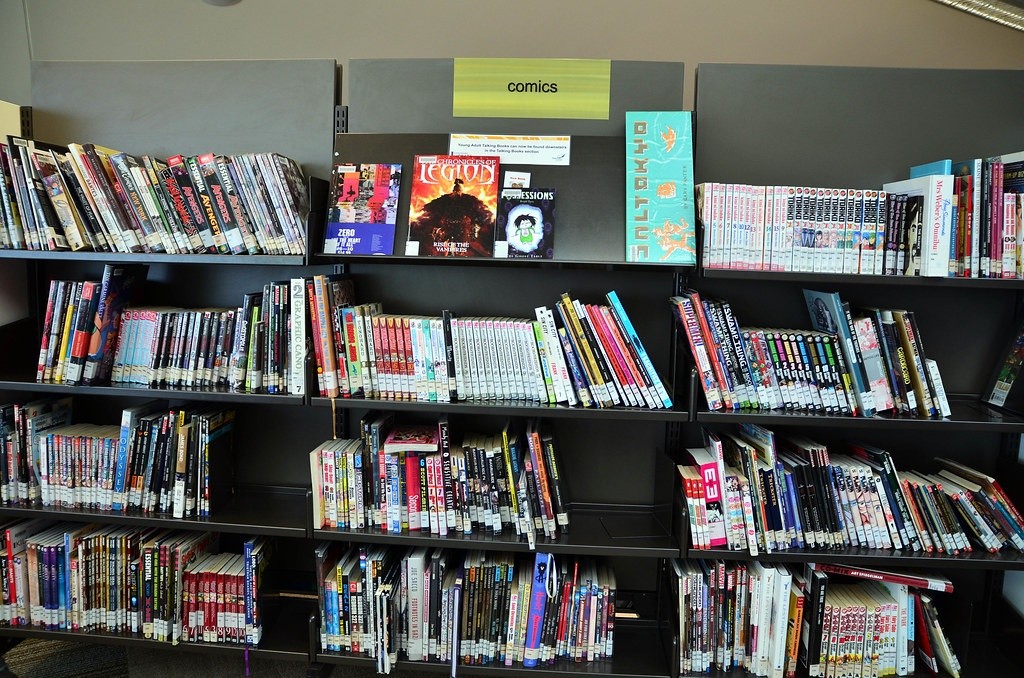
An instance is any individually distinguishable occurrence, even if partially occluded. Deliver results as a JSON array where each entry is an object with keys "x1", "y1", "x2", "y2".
[{"x1": 0, "y1": 110, "x2": 1024, "y2": 678}]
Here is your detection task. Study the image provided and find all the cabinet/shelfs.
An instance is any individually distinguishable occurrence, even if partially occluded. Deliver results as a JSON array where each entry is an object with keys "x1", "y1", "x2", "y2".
[
  {"x1": 676, "y1": 62, "x2": 1024, "y2": 678},
  {"x1": 304, "y1": 60, "x2": 689, "y2": 678},
  {"x1": 0, "y1": 58, "x2": 341, "y2": 678}
]
